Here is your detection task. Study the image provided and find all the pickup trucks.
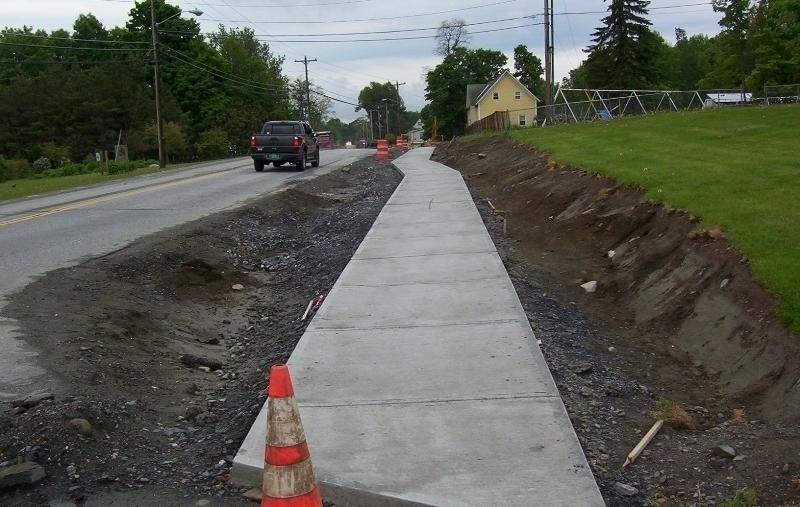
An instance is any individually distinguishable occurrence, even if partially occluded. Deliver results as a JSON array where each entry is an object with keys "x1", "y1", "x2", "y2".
[{"x1": 249, "y1": 120, "x2": 320, "y2": 173}]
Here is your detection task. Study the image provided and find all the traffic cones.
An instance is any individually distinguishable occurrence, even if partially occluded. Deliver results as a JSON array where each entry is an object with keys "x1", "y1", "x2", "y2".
[
  {"x1": 260, "y1": 363, "x2": 324, "y2": 507},
  {"x1": 396, "y1": 135, "x2": 408, "y2": 149},
  {"x1": 375, "y1": 139, "x2": 389, "y2": 160}
]
